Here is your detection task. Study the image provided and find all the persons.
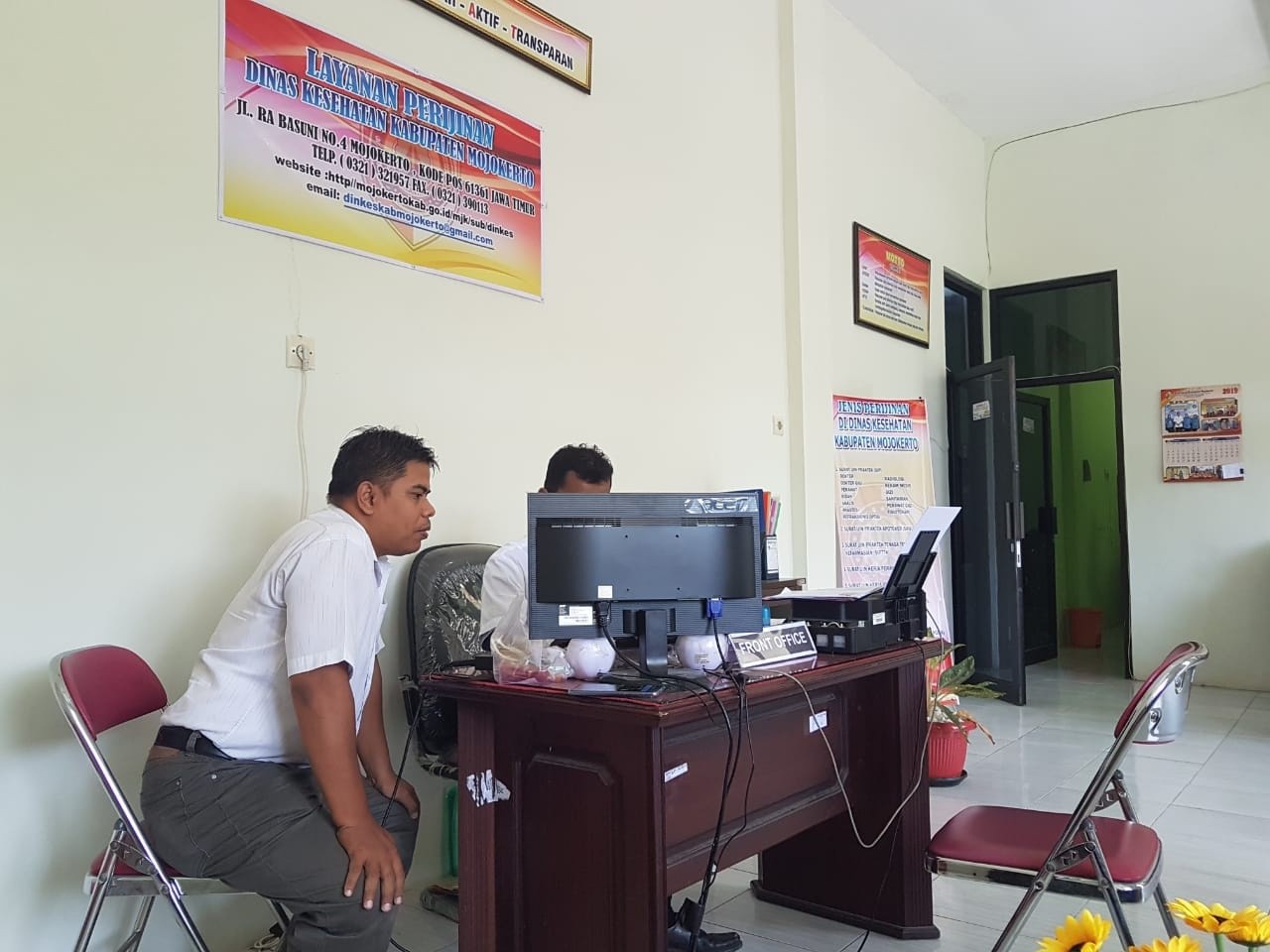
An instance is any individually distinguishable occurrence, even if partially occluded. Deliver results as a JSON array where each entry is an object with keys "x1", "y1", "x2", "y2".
[
  {"x1": 480, "y1": 444, "x2": 744, "y2": 952},
  {"x1": 140, "y1": 427, "x2": 440, "y2": 952}
]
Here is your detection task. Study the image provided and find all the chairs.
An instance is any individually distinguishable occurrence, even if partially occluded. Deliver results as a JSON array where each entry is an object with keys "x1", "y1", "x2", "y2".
[
  {"x1": 921, "y1": 638, "x2": 1209, "y2": 952},
  {"x1": 47, "y1": 645, "x2": 291, "y2": 952},
  {"x1": 404, "y1": 545, "x2": 501, "y2": 922}
]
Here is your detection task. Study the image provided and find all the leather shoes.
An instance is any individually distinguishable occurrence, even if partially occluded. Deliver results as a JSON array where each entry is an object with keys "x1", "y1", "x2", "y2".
[{"x1": 667, "y1": 911, "x2": 743, "y2": 952}]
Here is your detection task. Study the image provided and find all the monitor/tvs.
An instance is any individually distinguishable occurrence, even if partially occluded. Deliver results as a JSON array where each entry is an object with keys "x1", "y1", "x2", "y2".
[{"x1": 526, "y1": 491, "x2": 764, "y2": 684}]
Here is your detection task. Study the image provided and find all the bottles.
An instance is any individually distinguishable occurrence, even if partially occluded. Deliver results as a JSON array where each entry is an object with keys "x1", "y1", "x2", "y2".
[
  {"x1": 763, "y1": 605, "x2": 770, "y2": 626},
  {"x1": 675, "y1": 635, "x2": 728, "y2": 670},
  {"x1": 566, "y1": 638, "x2": 616, "y2": 678}
]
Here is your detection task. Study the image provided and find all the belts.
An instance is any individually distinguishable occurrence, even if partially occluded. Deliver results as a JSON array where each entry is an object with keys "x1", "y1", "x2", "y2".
[{"x1": 154, "y1": 726, "x2": 233, "y2": 761}]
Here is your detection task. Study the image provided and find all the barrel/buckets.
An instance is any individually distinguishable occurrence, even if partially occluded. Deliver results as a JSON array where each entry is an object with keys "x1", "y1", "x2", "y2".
[{"x1": 1066, "y1": 608, "x2": 1104, "y2": 647}]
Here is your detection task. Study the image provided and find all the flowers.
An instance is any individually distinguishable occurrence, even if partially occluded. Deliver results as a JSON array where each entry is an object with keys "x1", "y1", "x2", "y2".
[{"x1": 1034, "y1": 895, "x2": 1270, "y2": 952}]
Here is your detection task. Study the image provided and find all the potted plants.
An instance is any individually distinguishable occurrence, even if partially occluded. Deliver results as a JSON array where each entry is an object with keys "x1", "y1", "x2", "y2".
[{"x1": 926, "y1": 627, "x2": 1006, "y2": 787}]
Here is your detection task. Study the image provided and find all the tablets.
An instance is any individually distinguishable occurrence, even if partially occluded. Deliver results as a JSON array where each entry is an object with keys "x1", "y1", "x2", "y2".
[{"x1": 568, "y1": 682, "x2": 667, "y2": 696}]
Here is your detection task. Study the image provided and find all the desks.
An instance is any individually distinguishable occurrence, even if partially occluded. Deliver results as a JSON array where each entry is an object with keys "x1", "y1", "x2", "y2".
[{"x1": 418, "y1": 635, "x2": 948, "y2": 952}]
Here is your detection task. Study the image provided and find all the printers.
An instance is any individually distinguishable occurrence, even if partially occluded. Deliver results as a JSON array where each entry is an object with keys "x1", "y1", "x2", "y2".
[{"x1": 763, "y1": 530, "x2": 940, "y2": 655}]
